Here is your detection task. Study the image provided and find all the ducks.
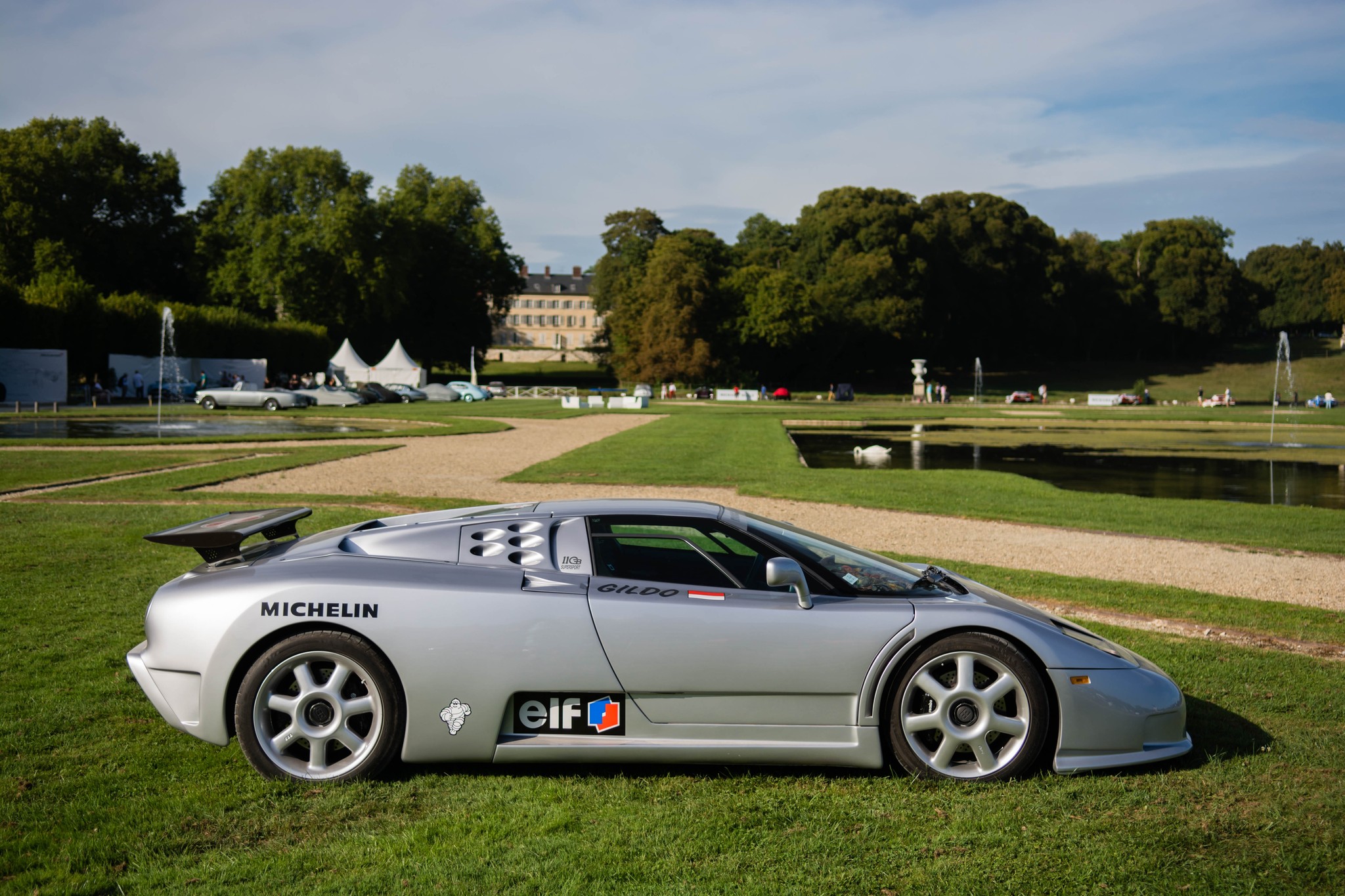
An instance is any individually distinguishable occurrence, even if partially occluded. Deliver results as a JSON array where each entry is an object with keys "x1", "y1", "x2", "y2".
[
  {"x1": 854, "y1": 453, "x2": 891, "y2": 466},
  {"x1": 854, "y1": 444, "x2": 893, "y2": 453}
]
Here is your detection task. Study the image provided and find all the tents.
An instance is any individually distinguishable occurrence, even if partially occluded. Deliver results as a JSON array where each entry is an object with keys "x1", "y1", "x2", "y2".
[
  {"x1": 369, "y1": 339, "x2": 427, "y2": 388},
  {"x1": 325, "y1": 337, "x2": 370, "y2": 385}
]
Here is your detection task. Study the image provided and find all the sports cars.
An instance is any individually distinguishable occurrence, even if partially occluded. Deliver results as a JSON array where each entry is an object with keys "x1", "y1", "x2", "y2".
[
  {"x1": 194, "y1": 381, "x2": 309, "y2": 411},
  {"x1": 295, "y1": 383, "x2": 363, "y2": 408},
  {"x1": 350, "y1": 382, "x2": 401, "y2": 405}
]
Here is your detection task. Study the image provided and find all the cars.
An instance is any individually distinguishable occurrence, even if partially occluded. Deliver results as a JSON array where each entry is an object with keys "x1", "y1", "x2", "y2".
[
  {"x1": 690, "y1": 386, "x2": 715, "y2": 399},
  {"x1": 148, "y1": 376, "x2": 199, "y2": 401},
  {"x1": 128, "y1": 498, "x2": 1194, "y2": 787},
  {"x1": 417, "y1": 382, "x2": 461, "y2": 397},
  {"x1": 477, "y1": 385, "x2": 494, "y2": 393},
  {"x1": 447, "y1": 381, "x2": 488, "y2": 401},
  {"x1": 488, "y1": 379, "x2": 507, "y2": 397},
  {"x1": 384, "y1": 383, "x2": 430, "y2": 401}
]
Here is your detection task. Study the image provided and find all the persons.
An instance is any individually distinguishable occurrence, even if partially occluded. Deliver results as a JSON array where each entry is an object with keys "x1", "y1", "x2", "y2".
[
  {"x1": 222, "y1": 370, "x2": 249, "y2": 387},
  {"x1": 661, "y1": 382, "x2": 676, "y2": 400},
  {"x1": 1277, "y1": 391, "x2": 1280, "y2": 406},
  {"x1": 827, "y1": 383, "x2": 836, "y2": 401},
  {"x1": 289, "y1": 374, "x2": 301, "y2": 390},
  {"x1": 199, "y1": 370, "x2": 207, "y2": 389},
  {"x1": 1038, "y1": 384, "x2": 1046, "y2": 403},
  {"x1": 121, "y1": 373, "x2": 128, "y2": 398},
  {"x1": 1197, "y1": 388, "x2": 1203, "y2": 406},
  {"x1": 132, "y1": 371, "x2": 144, "y2": 398},
  {"x1": 925, "y1": 382, "x2": 947, "y2": 403},
  {"x1": 263, "y1": 374, "x2": 282, "y2": 388},
  {"x1": 733, "y1": 385, "x2": 739, "y2": 400},
  {"x1": 1324, "y1": 390, "x2": 1332, "y2": 410},
  {"x1": 1293, "y1": 389, "x2": 1298, "y2": 406},
  {"x1": 1224, "y1": 386, "x2": 1230, "y2": 408},
  {"x1": 760, "y1": 384, "x2": 767, "y2": 400}
]
